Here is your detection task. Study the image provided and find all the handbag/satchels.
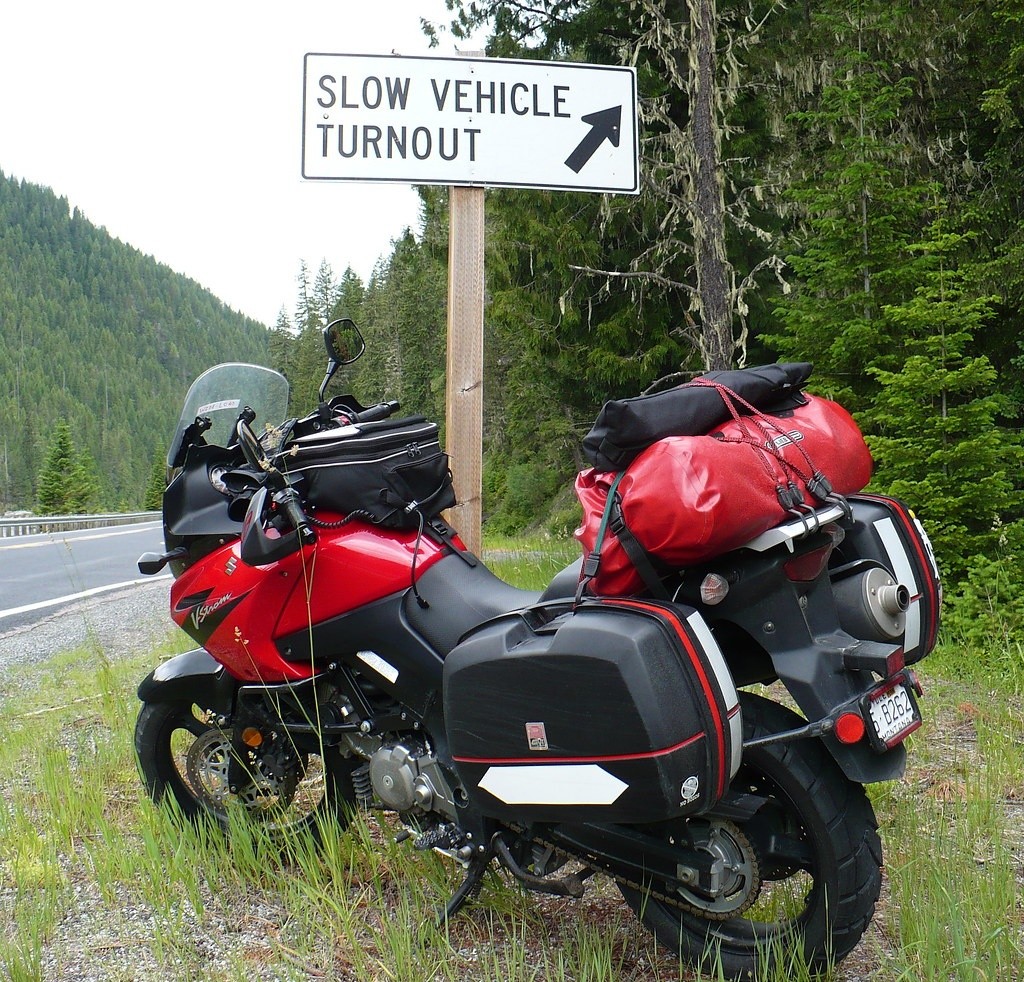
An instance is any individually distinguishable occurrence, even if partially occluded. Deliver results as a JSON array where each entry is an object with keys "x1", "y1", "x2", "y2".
[
  {"x1": 274, "y1": 415, "x2": 457, "y2": 529},
  {"x1": 573, "y1": 363, "x2": 813, "y2": 472},
  {"x1": 572, "y1": 392, "x2": 874, "y2": 598}
]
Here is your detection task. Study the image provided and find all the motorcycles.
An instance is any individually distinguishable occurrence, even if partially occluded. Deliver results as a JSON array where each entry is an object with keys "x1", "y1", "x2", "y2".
[{"x1": 129, "y1": 318, "x2": 944, "y2": 982}]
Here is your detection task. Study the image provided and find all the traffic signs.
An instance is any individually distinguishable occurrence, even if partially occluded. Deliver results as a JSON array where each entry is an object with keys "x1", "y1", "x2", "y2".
[{"x1": 301, "y1": 51, "x2": 643, "y2": 198}]
